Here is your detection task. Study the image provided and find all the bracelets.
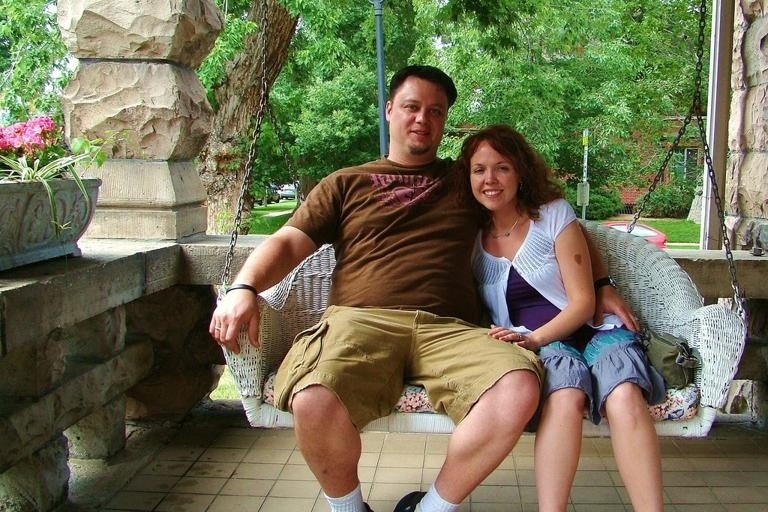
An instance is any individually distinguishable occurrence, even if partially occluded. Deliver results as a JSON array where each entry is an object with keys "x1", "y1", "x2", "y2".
[{"x1": 225, "y1": 284, "x2": 258, "y2": 298}]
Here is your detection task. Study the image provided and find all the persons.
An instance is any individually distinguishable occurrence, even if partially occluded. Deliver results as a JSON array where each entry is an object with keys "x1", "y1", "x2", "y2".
[
  {"x1": 209, "y1": 67, "x2": 643, "y2": 511},
  {"x1": 458, "y1": 125, "x2": 665, "y2": 511}
]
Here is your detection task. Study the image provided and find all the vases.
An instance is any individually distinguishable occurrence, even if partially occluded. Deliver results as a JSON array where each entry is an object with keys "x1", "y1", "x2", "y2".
[{"x1": 0, "y1": 174, "x2": 102, "y2": 271}]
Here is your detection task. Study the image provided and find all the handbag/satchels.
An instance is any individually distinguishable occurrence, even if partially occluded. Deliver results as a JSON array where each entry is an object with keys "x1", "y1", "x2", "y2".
[{"x1": 639, "y1": 326, "x2": 700, "y2": 390}]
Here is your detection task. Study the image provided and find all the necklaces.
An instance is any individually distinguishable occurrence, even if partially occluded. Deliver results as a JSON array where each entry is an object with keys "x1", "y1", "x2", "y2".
[{"x1": 487, "y1": 217, "x2": 520, "y2": 238}]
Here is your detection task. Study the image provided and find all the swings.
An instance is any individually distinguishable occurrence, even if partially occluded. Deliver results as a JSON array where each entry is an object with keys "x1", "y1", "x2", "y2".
[{"x1": 217, "y1": 1, "x2": 748, "y2": 438}]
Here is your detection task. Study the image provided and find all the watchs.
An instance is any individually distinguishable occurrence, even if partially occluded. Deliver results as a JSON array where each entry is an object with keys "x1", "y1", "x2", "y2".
[{"x1": 595, "y1": 274, "x2": 618, "y2": 293}]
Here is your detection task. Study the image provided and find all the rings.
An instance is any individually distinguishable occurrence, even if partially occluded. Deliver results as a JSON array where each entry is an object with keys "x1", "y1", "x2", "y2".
[
  {"x1": 633, "y1": 316, "x2": 641, "y2": 322},
  {"x1": 517, "y1": 331, "x2": 523, "y2": 340},
  {"x1": 213, "y1": 326, "x2": 221, "y2": 330}
]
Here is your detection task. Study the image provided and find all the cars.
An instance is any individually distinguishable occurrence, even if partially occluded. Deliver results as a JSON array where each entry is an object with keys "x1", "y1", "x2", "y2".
[
  {"x1": 596, "y1": 220, "x2": 668, "y2": 252},
  {"x1": 250, "y1": 178, "x2": 299, "y2": 207}
]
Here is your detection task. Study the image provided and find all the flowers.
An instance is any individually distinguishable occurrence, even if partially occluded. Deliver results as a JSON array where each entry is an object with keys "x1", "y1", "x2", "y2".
[{"x1": 1, "y1": 110, "x2": 125, "y2": 240}]
